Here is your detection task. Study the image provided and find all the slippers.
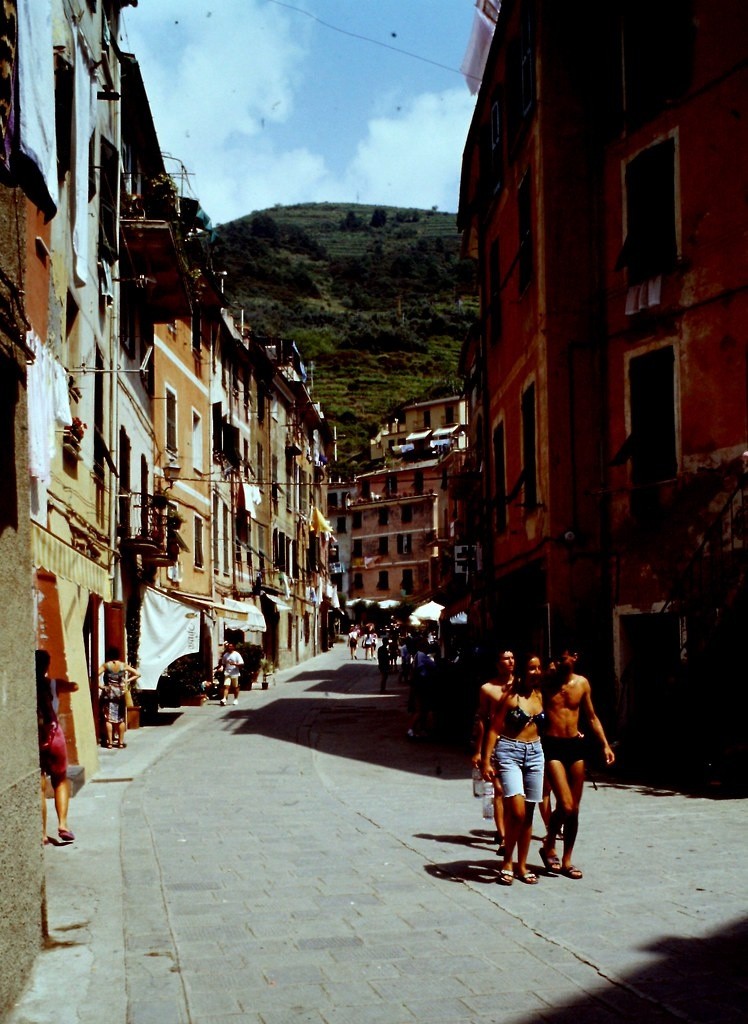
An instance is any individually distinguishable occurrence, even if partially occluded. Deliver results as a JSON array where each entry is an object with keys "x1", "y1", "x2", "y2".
[
  {"x1": 44, "y1": 840, "x2": 49, "y2": 845},
  {"x1": 561, "y1": 866, "x2": 583, "y2": 880},
  {"x1": 60, "y1": 831, "x2": 75, "y2": 840},
  {"x1": 538, "y1": 847, "x2": 561, "y2": 874},
  {"x1": 515, "y1": 868, "x2": 538, "y2": 884},
  {"x1": 498, "y1": 869, "x2": 514, "y2": 886}
]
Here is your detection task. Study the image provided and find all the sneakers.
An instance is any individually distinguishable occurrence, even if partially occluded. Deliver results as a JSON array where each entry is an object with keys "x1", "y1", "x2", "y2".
[
  {"x1": 219, "y1": 698, "x2": 227, "y2": 706},
  {"x1": 233, "y1": 699, "x2": 238, "y2": 705}
]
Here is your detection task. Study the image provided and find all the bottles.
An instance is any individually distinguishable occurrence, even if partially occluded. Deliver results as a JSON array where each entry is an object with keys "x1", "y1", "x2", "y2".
[
  {"x1": 481, "y1": 780, "x2": 496, "y2": 821},
  {"x1": 472, "y1": 764, "x2": 484, "y2": 800}
]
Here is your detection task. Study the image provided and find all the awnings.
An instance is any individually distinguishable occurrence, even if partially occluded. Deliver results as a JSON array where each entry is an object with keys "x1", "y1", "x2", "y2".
[
  {"x1": 390, "y1": 424, "x2": 462, "y2": 452},
  {"x1": 266, "y1": 594, "x2": 292, "y2": 613},
  {"x1": 157, "y1": 586, "x2": 247, "y2": 621},
  {"x1": 221, "y1": 597, "x2": 268, "y2": 633}
]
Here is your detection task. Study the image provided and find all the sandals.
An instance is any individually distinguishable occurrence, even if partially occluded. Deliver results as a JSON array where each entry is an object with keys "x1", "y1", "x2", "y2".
[
  {"x1": 120, "y1": 743, "x2": 127, "y2": 749},
  {"x1": 106, "y1": 743, "x2": 114, "y2": 749}
]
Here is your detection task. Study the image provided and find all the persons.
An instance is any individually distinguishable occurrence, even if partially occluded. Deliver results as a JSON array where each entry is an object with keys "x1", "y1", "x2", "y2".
[
  {"x1": 35, "y1": 649, "x2": 80, "y2": 843},
  {"x1": 95, "y1": 646, "x2": 140, "y2": 749},
  {"x1": 471, "y1": 644, "x2": 614, "y2": 885},
  {"x1": 213, "y1": 641, "x2": 245, "y2": 707},
  {"x1": 344, "y1": 625, "x2": 377, "y2": 661},
  {"x1": 377, "y1": 633, "x2": 442, "y2": 693}
]
